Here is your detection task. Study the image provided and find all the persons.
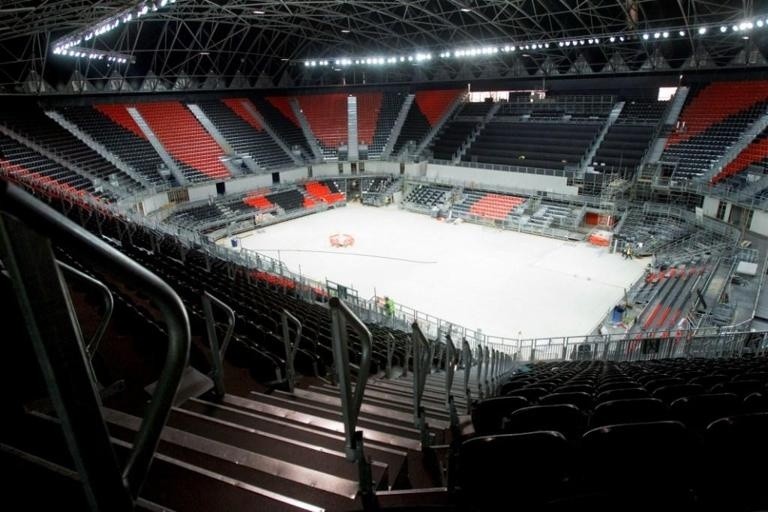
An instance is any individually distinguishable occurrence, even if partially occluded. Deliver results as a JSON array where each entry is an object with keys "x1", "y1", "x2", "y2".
[
  {"x1": 378, "y1": 297, "x2": 395, "y2": 325},
  {"x1": 623, "y1": 243, "x2": 642, "y2": 260}
]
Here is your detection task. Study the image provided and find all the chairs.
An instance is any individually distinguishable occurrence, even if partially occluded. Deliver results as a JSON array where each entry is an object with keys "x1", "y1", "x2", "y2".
[
  {"x1": 1, "y1": 171, "x2": 481, "y2": 511},
  {"x1": 444, "y1": 354, "x2": 768, "y2": 511}
]
